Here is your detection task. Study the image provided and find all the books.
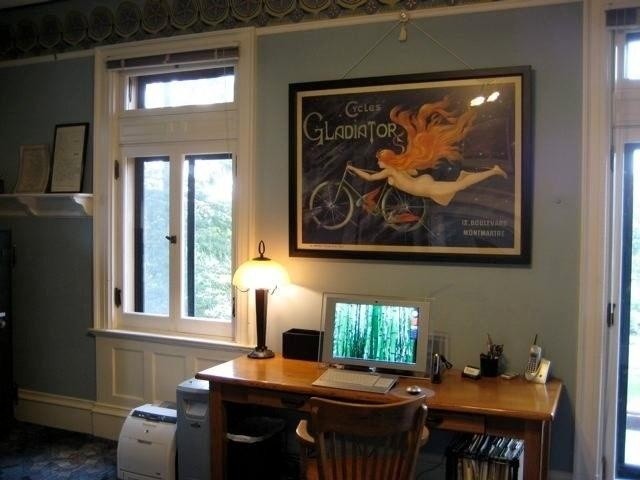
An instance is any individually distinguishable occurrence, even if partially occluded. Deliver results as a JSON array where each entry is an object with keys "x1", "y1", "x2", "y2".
[{"x1": 457, "y1": 433, "x2": 523, "y2": 479}]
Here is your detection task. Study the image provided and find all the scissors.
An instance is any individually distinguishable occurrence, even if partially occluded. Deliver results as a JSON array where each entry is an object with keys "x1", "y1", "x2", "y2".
[{"x1": 494, "y1": 342, "x2": 504, "y2": 360}]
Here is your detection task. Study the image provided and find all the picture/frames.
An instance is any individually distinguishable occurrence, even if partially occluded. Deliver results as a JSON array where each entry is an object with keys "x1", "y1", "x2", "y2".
[
  {"x1": 46, "y1": 122, "x2": 90, "y2": 192},
  {"x1": 289, "y1": 66, "x2": 533, "y2": 265}
]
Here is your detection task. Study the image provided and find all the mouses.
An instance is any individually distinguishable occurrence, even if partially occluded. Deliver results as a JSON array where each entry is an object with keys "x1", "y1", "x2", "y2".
[{"x1": 406, "y1": 385, "x2": 421, "y2": 393}]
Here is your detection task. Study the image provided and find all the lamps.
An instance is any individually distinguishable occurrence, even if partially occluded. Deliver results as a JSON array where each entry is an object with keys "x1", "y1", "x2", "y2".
[{"x1": 233, "y1": 239, "x2": 289, "y2": 359}]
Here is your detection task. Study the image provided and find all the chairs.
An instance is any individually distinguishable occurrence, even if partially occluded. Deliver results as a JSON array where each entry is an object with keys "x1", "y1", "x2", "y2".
[{"x1": 295, "y1": 394, "x2": 427, "y2": 476}]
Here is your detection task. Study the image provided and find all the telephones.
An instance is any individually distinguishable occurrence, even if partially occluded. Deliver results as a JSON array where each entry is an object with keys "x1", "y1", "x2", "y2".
[{"x1": 525, "y1": 333, "x2": 551, "y2": 384}]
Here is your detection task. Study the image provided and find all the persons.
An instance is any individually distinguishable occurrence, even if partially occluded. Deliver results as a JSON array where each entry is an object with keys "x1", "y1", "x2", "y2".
[{"x1": 347, "y1": 97, "x2": 507, "y2": 207}]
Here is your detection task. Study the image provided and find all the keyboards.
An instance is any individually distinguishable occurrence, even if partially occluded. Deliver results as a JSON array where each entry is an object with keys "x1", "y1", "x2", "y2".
[{"x1": 312, "y1": 368, "x2": 400, "y2": 394}]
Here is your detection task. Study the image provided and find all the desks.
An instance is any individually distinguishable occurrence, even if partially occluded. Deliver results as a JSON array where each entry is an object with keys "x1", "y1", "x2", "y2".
[{"x1": 195, "y1": 351, "x2": 561, "y2": 476}]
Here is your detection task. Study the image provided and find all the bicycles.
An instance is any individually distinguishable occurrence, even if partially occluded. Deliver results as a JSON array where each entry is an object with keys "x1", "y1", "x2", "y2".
[{"x1": 310, "y1": 160, "x2": 428, "y2": 232}]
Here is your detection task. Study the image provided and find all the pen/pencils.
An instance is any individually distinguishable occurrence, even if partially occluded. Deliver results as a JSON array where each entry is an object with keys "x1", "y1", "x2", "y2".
[{"x1": 487, "y1": 334, "x2": 491, "y2": 352}]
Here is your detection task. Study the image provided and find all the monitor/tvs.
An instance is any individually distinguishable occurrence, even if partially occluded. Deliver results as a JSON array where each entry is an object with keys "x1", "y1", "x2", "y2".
[{"x1": 318, "y1": 291, "x2": 434, "y2": 373}]
